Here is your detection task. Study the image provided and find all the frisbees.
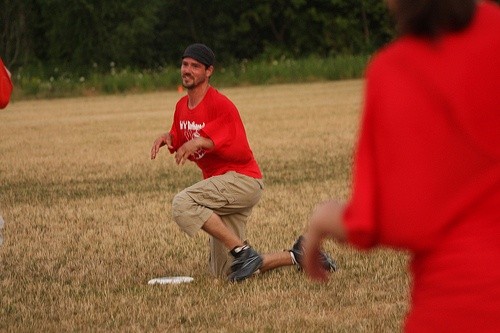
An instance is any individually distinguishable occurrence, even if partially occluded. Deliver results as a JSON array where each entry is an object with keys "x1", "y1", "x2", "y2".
[{"x1": 147, "y1": 275, "x2": 194, "y2": 286}]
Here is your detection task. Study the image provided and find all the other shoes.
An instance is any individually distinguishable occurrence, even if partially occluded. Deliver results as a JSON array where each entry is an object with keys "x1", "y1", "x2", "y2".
[
  {"x1": 227, "y1": 239, "x2": 262, "y2": 283},
  {"x1": 288, "y1": 235, "x2": 336, "y2": 274}
]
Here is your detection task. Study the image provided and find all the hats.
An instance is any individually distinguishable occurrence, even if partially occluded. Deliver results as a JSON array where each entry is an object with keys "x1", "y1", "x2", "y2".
[{"x1": 181, "y1": 43, "x2": 214, "y2": 65}]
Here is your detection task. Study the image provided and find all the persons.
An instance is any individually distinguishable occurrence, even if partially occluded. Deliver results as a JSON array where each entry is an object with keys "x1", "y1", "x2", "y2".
[
  {"x1": 150, "y1": 43, "x2": 336, "y2": 282},
  {"x1": 299, "y1": 0, "x2": 500, "y2": 333}
]
